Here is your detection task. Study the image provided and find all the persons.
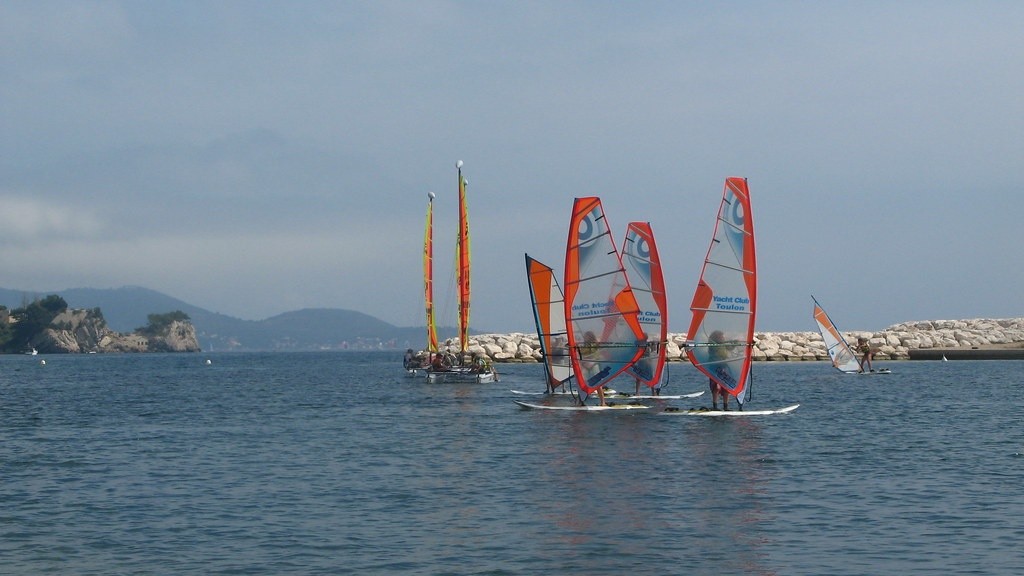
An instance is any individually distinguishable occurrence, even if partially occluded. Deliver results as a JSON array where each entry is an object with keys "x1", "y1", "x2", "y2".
[
  {"x1": 403, "y1": 349, "x2": 464, "y2": 371},
  {"x1": 579, "y1": 332, "x2": 607, "y2": 406},
  {"x1": 540, "y1": 337, "x2": 567, "y2": 394},
  {"x1": 851, "y1": 338, "x2": 872, "y2": 371},
  {"x1": 633, "y1": 333, "x2": 658, "y2": 395},
  {"x1": 707, "y1": 331, "x2": 741, "y2": 410},
  {"x1": 467, "y1": 353, "x2": 487, "y2": 373}
]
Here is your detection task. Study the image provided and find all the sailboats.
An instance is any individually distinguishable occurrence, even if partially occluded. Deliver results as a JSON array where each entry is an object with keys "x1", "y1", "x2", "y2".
[
  {"x1": 425, "y1": 159, "x2": 498, "y2": 383},
  {"x1": 509, "y1": 253, "x2": 616, "y2": 395},
  {"x1": 600, "y1": 219, "x2": 705, "y2": 400},
  {"x1": 657, "y1": 178, "x2": 801, "y2": 415},
  {"x1": 510, "y1": 197, "x2": 655, "y2": 411},
  {"x1": 403, "y1": 191, "x2": 439, "y2": 376},
  {"x1": 810, "y1": 295, "x2": 892, "y2": 374}
]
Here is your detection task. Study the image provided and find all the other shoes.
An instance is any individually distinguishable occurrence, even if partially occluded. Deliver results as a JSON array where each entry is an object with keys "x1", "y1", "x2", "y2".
[
  {"x1": 859, "y1": 370, "x2": 864, "y2": 373},
  {"x1": 724, "y1": 408, "x2": 732, "y2": 411},
  {"x1": 713, "y1": 407, "x2": 722, "y2": 411},
  {"x1": 869, "y1": 370, "x2": 875, "y2": 372}
]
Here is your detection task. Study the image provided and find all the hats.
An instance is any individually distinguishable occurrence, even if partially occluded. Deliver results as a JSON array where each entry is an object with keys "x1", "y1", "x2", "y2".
[
  {"x1": 474, "y1": 356, "x2": 480, "y2": 363},
  {"x1": 471, "y1": 352, "x2": 476, "y2": 360},
  {"x1": 436, "y1": 353, "x2": 444, "y2": 357}
]
